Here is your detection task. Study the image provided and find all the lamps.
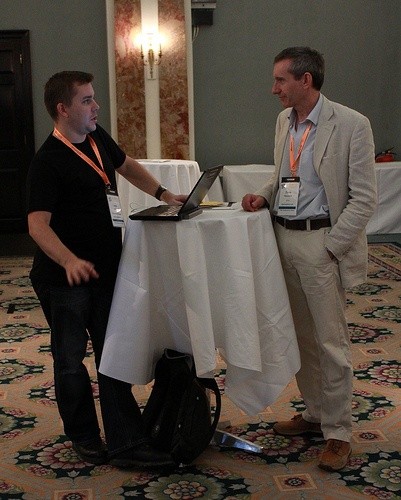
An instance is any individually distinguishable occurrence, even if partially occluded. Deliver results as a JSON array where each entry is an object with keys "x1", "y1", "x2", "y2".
[{"x1": 137, "y1": 28, "x2": 165, "y2": 80}]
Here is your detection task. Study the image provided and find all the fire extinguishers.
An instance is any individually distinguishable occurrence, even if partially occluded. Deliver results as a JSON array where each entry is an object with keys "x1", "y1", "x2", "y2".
[{"x1": 375, "y1": 146, "x2": 398, "y2": 163}]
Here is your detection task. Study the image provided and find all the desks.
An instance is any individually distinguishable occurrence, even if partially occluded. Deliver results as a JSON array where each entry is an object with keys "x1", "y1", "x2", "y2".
[
  {"x1": 124, "y1": 201, "x2": 273, "y2": 457},
  {"x1": 220, "y1": 165, "x2": 278, "y2": 202},
  {"x1": 115, "y1": 157, "x2": 201, "y2": 216},
  {"x1": 365, "y1": 162, "x2": 401, "y2": 244}
]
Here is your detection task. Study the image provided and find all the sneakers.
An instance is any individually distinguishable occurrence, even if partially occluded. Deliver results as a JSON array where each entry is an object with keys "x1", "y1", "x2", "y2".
[
  {"x1": 319, "y1": 439, "x2": 353, "y2": 472},
  {"x1": 273, "y1": 413, "x2": 322, "y2": 435},
  {"x1": 72, "y1": 437, "x2": 110, "y2": 465}
]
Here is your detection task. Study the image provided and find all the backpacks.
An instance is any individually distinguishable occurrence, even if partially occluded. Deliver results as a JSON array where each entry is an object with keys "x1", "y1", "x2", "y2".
[{"x1": 114, "y1": 348, "x2": 222, "y2": 472}]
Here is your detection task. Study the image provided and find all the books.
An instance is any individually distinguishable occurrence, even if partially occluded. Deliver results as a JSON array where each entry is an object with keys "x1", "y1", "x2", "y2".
[{"x1": 199, "y1": 201, "x2": 242, "y2": 211}]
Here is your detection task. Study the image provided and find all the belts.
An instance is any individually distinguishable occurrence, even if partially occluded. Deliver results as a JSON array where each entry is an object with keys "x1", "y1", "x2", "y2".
[{"x1": 273, "y1": 214, "x2": 331, "y2": 231}]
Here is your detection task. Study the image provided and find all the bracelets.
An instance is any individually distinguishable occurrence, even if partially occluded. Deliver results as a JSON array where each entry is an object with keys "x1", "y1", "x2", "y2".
[{"x1": 154, "y1": 184, "x2": 168, "y2": 201}]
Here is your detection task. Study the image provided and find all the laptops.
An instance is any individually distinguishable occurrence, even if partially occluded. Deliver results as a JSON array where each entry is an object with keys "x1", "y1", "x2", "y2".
[{"x1": 129, "y1": 164, "x2": 224, "y2": 221}]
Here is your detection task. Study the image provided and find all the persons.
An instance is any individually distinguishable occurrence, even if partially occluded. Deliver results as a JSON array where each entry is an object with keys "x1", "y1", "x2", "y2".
[
  {"x1": 240, "y1": 45, "x2": 379, "y2": 473},
  {"x1": 24, "y1": 71, "x2": 189, "y2": 468}
]
